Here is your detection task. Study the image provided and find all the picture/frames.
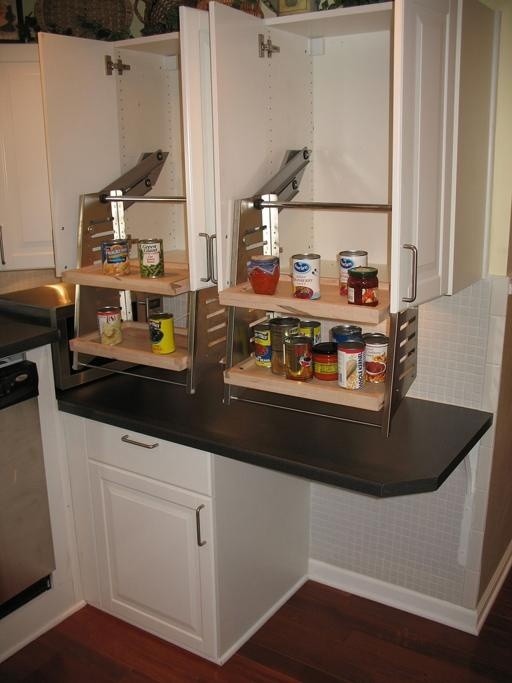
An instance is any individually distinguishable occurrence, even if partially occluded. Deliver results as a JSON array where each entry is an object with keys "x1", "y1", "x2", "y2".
[{"x1": 0, "y1": 0, "x2": 25, "y2": 43}]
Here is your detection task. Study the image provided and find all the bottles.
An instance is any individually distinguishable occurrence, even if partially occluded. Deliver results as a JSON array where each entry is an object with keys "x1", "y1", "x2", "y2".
[{"x1": 247, "y1": 254, "x2": 280, "y2": 294}]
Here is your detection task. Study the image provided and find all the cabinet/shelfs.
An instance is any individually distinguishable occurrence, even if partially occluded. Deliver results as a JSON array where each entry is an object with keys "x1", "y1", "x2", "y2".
[
  {"x1": 37, "y1": 4, "x2": 224, "y2": 396},
  {"x1": 56, "y1": 403, "x2": 310, "y2": 665},
  {"x1": 0, "y1": 41, "x2": 55, "y2": 268},
  {"x1": 209, "y1": 1, "x2": 498, "y2": 438}
]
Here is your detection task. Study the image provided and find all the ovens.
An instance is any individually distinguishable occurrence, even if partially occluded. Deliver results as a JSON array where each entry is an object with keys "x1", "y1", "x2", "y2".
[{"x1": 0, "y1": 359, "x2": 57, "y2": 618}]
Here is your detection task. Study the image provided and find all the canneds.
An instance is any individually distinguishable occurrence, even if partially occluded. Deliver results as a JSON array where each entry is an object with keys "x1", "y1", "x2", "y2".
[
  {"x1": 337, "y1": 251, "x2": 368, "y2": 297},
  {"x1": 137, "y1": 238, "x2": 165, "y2": 278},
  {"x1": 251, "y1": 316, "x2": 390, "y2": 391},
  {"x1": 95, "y1": 305, "x2": 123, "y2": 346},
  {"x1": 291, "y1": 253, "x2": 321, "y2": 300},
  {"x1": 346, "y1": 266, "x2": 380, "y2": 307},
  {"x1": 100, "y1": 238, "x2": 130, "y2": 277},
  {"x1": 147, "y1": 312, "x2": 176, "y2": 355}
]
